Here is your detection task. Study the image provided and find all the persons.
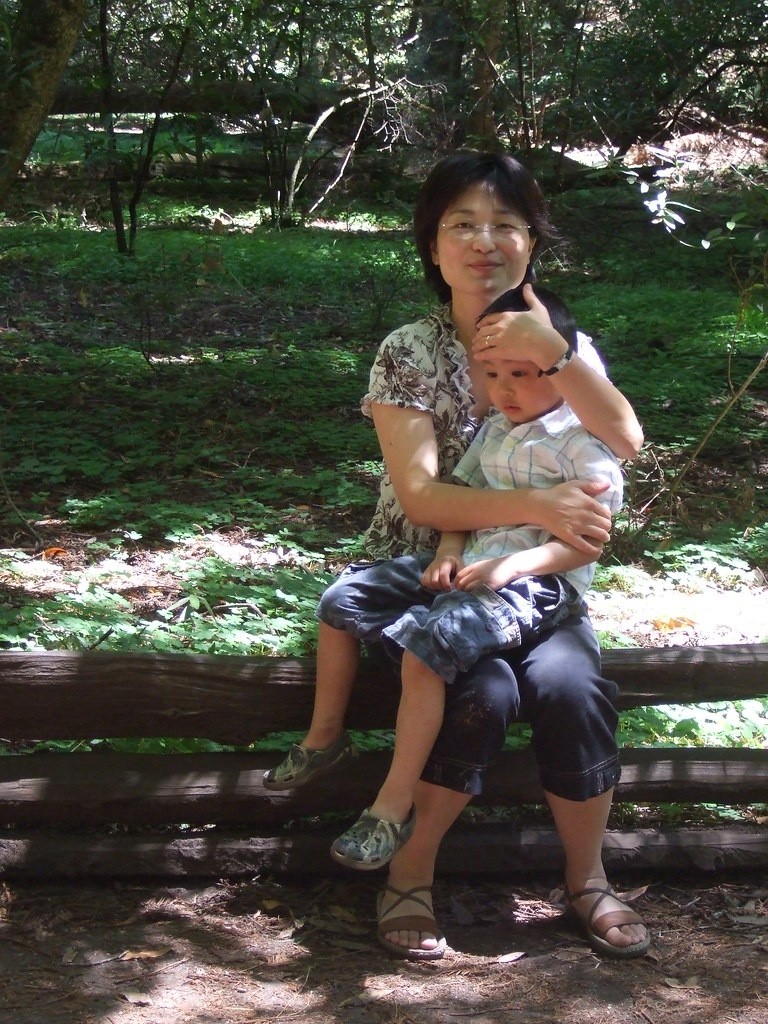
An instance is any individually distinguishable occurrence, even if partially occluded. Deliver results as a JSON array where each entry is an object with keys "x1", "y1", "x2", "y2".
[
  {"x1": 356, "y1": 146, "x2": 653, "y2": 958},
  {"x1": 264, "y1": 285, "x2": 624, "y2": 872}
]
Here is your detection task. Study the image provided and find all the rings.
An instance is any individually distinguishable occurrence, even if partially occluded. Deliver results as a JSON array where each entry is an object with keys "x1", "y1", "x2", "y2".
[{"x1": 485, "y1": 335, "x2": 490, "y2": 347}]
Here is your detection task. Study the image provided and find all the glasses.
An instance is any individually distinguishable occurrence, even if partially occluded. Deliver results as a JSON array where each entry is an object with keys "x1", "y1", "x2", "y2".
[{"x1": 435, "y1": 218, "x2": 531, "y2": 240}]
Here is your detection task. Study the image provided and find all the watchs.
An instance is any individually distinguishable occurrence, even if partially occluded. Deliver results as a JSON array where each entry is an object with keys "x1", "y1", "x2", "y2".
[{"x1": 543, "y1": 345, "x2": 575, "y2": 376}]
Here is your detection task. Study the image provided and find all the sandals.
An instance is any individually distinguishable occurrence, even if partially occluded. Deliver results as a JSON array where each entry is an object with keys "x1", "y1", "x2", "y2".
[
  {"x1": 329, "y1": 801, "x2": 416, "y2": 870},
  {"x1": 375, "y1": 878, "x2": 446, "y2": 959},
  {"x1": 263, "y1": 730, "x2": 351, "y2": 791},
  {"x1": 565, "y1": 881, "x2": 650, "y2": 958}
]
